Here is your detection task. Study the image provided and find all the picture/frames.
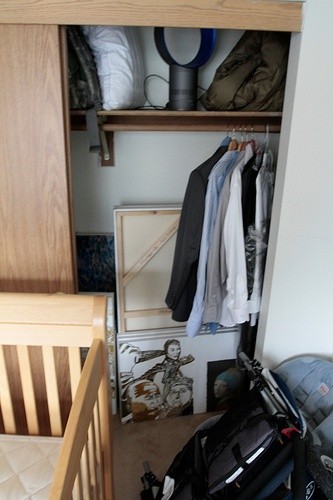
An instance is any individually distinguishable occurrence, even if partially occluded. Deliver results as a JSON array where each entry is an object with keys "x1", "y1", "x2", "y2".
[{"x1": 116, "y1": 324, "x2": 242, "y2": 425}]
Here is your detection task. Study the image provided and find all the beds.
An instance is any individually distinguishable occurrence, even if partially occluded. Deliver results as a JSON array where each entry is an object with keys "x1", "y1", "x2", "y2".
[{"x1": 0, "y1": 291, "x2": 116, "y2": 499}]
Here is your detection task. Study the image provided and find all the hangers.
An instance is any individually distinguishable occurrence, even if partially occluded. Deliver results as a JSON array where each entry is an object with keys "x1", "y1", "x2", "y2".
[{"x1": 225, "y1": 119, "x2": 274, "y2": 173}]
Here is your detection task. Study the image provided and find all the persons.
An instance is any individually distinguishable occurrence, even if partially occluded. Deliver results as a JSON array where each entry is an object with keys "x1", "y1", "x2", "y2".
[
  {"x1": 151, "y1": 339, "x2": 194, "y2": 415},
  {"x1": 213, "y1": 367, "x2": 242, "y2": 409}
]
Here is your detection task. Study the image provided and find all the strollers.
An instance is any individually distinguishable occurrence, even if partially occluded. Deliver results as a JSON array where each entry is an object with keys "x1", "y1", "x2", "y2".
[{"x1": 141, "y1": 353, "x2": 333, "y2": 500}]
